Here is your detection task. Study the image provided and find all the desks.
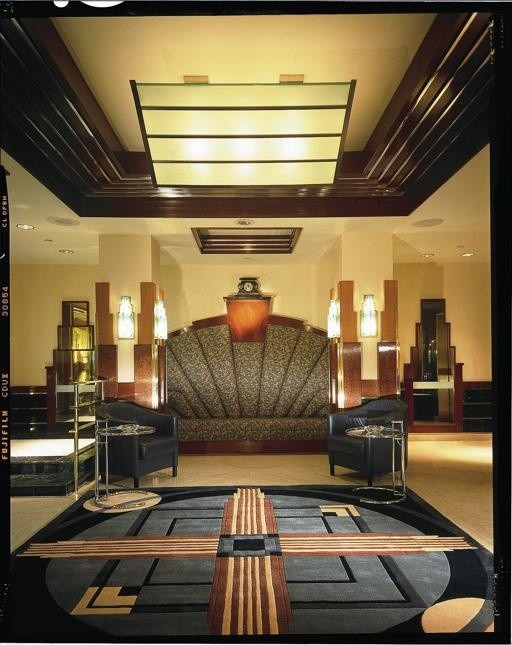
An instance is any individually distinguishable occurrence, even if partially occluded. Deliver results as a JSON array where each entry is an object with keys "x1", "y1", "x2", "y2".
[
  {"x1": 81, "y1": 420, "x2": 163, "y2": 514},
  {"x1": 342, "y1": 420, "x2": 407, "y2": 506}
]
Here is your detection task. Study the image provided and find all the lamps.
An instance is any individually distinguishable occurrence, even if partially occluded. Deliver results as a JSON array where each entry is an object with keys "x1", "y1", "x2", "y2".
[
  {"x1": 154, "y1": 300, "x2": 169, "y2": 341},
  {"x1": 132, "y1": 73, "x2": 358, "y2": 191},
  {"x1": 360, "y1": 294, "x2": 378, "y2": 338},
  {"x1": 116, "y1": 296, "x2": 135, "y2": 341},
  {"x1": 325, "y1": 298, "x2": 340, "y2": 343}
]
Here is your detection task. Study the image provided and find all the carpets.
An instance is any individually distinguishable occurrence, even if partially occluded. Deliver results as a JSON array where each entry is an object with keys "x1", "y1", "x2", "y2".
[{"x1": 11, "y1": 484, "x2": 494, "y2": 635}]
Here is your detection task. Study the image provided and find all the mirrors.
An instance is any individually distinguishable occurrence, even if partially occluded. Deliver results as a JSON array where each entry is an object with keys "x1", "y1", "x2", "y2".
[
  {"x1": 402, "y1": 298, "x2": 464, "y2": 434},
  {"x1": 46, "y1": 300, "x2": 96, "y2": 435}
]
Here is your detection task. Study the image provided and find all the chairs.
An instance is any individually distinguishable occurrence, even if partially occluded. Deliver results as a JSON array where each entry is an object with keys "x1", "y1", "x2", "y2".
[
  {"x1": 96, "y1": 398, "x2": 181, "y2": 488},
  {"x1": 327, "y1": 398, "x2": 409, "y2": 486}
]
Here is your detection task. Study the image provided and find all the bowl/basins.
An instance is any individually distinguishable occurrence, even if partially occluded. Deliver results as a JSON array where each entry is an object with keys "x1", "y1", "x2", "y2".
[
  {"x1": 365, "y1": 425, "x2": 383, "y2": 434},
  {"x1": 119, "y1": 424, "x2": 139, "y2": 432}
]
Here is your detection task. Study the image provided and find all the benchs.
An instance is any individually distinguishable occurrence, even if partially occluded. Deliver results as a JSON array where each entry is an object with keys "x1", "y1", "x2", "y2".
[{"x1": 164, "y1": 299, "x2": 333, "y2": 457}]
